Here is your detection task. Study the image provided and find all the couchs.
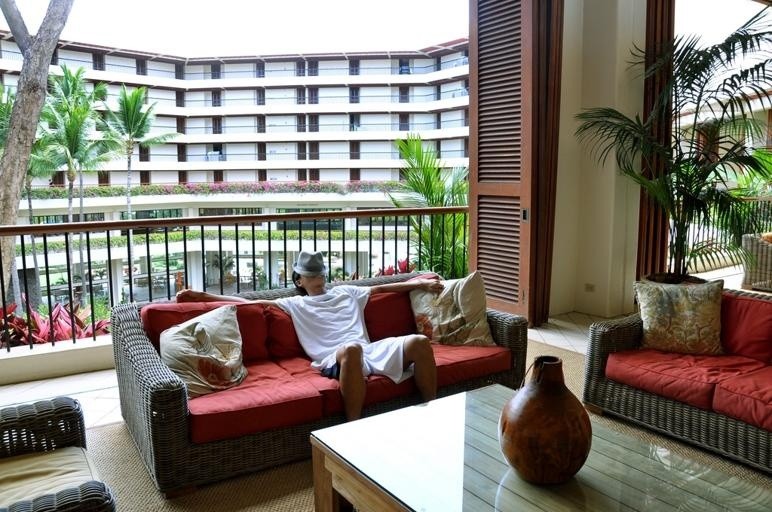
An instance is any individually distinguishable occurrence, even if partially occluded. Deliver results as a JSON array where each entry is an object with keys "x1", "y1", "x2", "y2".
[
  {"x1": 110, "y1": 271, "x2": 529, "y2": 500},
  {"x1": 0, "y1": 397, "x2": 117, "y2": 512},
  {"x1": 741, "y1": 232, "x2": 772, "y2": 291},
  {"x1": 582, "y1": 287, "x2": 771, "y2": 475}
]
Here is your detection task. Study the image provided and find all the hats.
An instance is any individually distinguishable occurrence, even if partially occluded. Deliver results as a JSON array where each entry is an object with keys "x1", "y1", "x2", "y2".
[{"x1": 291, "y1": 251, "x2": 328, "y2": 277}]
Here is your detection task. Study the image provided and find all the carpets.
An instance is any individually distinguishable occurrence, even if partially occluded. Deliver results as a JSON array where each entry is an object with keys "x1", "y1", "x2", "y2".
[{"x1": 86, "y1": 338, "x2": 772, "y2": 512}]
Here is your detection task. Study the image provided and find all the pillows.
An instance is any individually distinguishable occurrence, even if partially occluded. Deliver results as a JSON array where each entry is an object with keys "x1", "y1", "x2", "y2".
[
  {"x1": 634, "y1": 279, "x2": 725, "y2": 355},
  {"x1": 409, "y1": 269, "x2": 496, "y2": 347},
  {"x1": 156, "y1": 304, "x2": 249, "y2": 398}
]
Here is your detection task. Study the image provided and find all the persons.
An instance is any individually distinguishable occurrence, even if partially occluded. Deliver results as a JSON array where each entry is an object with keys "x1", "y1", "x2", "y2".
[{"x1": 175, "y1": 251, "x2": 445, "y2": 421}]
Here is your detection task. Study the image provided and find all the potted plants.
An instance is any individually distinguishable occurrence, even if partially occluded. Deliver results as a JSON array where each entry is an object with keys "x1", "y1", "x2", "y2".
[{"x1": 573, "y1": 5, "x2": 772, "y2": 284}]
